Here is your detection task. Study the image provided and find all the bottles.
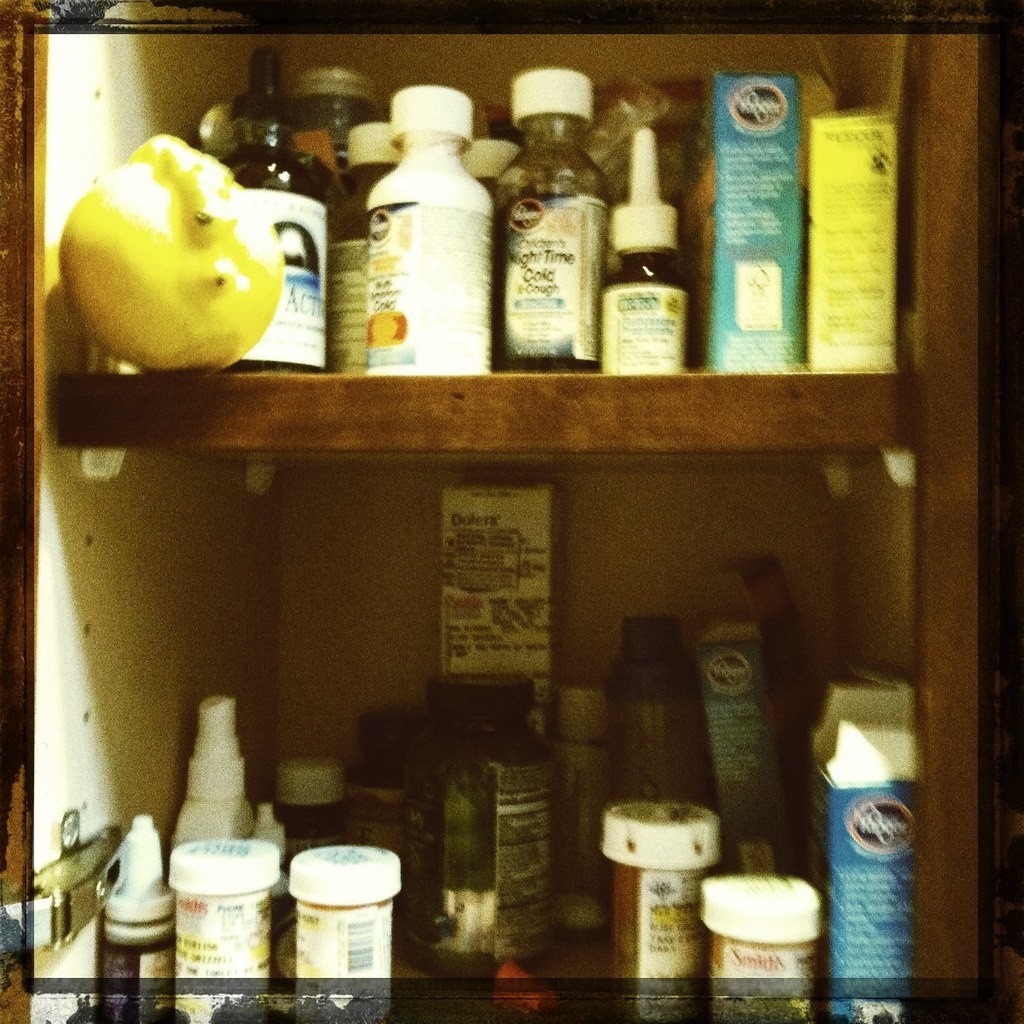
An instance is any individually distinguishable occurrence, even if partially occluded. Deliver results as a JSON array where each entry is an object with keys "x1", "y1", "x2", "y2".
[
  {"x1": 273, "y1": 752, "x2": 350, "y2": 1022},
  {"x1": 176, "y1": 694, "x2": 250, "y2": 851},
  {"x1": 611, "y1": 612, "x2": 706, "y2": 805},
  {"x1": 398, "y1": 675, "x2": 549, "y2": 976},
  {"x1": 94, "y1": 814, "x2": 173, "y2": 1022},
  {"x1": 349, "y1": 717, "x2": 417, "y2": 854},
  {"x1": 549, "y1": 683, "x2": 613, "y2": 938},
  {"x1": 209, "y1": 46, "x2": 688, "y2": 375}
]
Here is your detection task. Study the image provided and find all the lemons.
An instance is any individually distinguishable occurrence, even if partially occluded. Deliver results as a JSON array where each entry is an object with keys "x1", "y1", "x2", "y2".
[{"x1": 59, "y1": 132, "x2": 285, "y2": 373}]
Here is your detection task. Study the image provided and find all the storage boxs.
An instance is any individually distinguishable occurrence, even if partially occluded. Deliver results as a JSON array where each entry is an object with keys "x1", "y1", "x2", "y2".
[
  {"x1": 684, "y1": 68, "x2": 808, "y2": 381},
  {"x1": 804, "y1": 105, "x2": 898, "y2": 376}
]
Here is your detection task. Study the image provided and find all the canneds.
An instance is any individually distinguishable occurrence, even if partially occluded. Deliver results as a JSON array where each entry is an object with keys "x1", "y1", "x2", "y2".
[
  {"x1": 167, "y1": 839, "x2": 402, "y2": 1024},
  {"x1": 597, "y1": 800, "x2": 821, "y2": 1024}
]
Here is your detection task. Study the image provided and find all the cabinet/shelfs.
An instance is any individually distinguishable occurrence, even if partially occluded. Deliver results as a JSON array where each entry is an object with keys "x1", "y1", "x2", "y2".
[{"x1": 5, "y1": 0, "x2": 994, "y2": 1012}]
